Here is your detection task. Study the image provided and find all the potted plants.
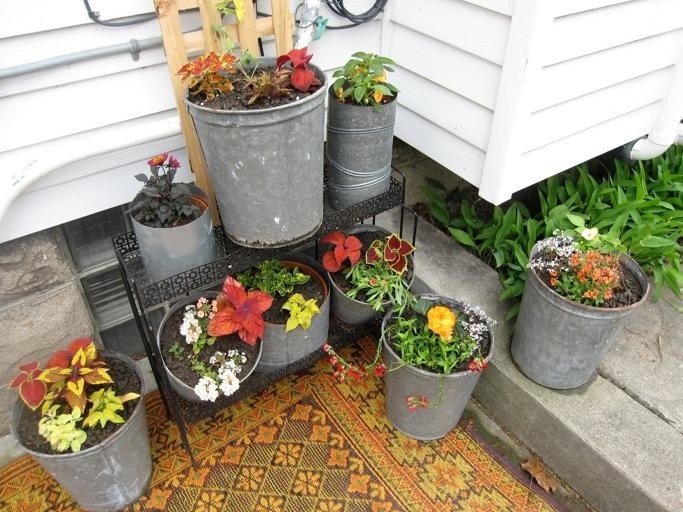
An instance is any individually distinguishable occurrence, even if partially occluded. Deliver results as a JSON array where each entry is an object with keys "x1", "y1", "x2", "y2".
[
  {"x1": 320, "y1": 223, "x2": 415, "y2": 327},
  {"x1": 3, "y1": 338, "x2": 151, "y2": 511},
  {"x1": 326, "y1": 52, "x2": 399, "y2": 212},
  {"x1": 234, "y1": 259, "x2": 330, "y2": 374}
]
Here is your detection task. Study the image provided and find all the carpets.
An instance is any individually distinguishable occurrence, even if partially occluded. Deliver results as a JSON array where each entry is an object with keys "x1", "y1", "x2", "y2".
[{"x1": 0, "y1": 320, "x2": 593, "y2": 512}]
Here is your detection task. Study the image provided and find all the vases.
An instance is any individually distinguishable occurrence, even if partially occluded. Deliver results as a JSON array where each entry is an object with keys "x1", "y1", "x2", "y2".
[
  {"x1": 183, "y1": 56, "x2": 323, "y2": 249},
  {"x1": 509, "y1": 237, "x2": 650, "y2": 391},
  {"x1": 128, "y1": 183, "x2": 218, "y2": 301},
  {"x1": 154, "y1": 291, "x2": 263, "y2": 403},
  {"x1": 380, "y1": 292, "x2": 494, "y2": 442}
]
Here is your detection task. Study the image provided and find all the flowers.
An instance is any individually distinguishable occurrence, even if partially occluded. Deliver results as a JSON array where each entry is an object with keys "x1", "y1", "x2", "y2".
[
  {"x1": 322, "y1": 294, "x2": 498, "y2": 410},
  {"x1": 176, "y1": 0, "x2": 320, "y2": 107},
  {"x1": 525, "y1": 227, "x2": 627, "y2": 306},
  {"x1": 165, "y1": 277, "x2": 272, "y2": 402},
  {"x1": 123, "y1": 152, "x2": 201, "y2": 229}
]
